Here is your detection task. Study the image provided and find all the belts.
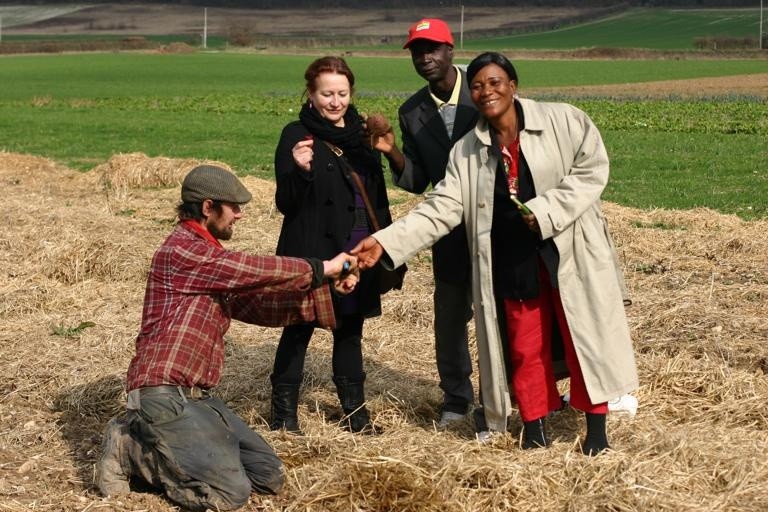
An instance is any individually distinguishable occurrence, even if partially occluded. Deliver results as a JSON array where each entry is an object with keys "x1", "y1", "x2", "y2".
[{"x1": 135, "y1": 384, "x2": 209, "y2": 402}]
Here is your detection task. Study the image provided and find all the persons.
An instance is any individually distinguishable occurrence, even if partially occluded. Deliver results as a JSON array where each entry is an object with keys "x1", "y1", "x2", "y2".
[
  {"x1": 361, "y1": 18, "x2": 490, "y2": 444},
  {"x1": 269, "y1": 55, "x2": 409, "y2": 437},
  {"x1": 351, "y1": 52, "x2": 639, "y2": 456},
  {"x1": 99, "y1": 165, "x2": 360, "y2": 510}
]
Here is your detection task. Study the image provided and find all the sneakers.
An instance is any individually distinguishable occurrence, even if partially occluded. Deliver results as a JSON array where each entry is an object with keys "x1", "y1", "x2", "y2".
[
  {"x1": 475, "y1": 427, "x2": 498, "y2": 446},
  {"x1": 94, "y1": 414, "x2": 134, "y2": 497},
  {"x1": 440, "y1": 409, "x2": 465, "y2": 429}
]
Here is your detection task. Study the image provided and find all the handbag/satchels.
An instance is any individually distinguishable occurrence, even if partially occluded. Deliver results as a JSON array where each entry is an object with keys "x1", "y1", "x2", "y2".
[{"x1": 377, "y1": 254, "x2": 409, "y2": 295}]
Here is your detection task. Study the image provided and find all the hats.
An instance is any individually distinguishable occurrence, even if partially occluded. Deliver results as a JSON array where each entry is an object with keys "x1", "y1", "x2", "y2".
[
  {"x1": 180, "y1": 164, "x2": 253, "y2": 207},
  {"x1": 402, "y1": 17, "x2": 455, "y2": 51}
]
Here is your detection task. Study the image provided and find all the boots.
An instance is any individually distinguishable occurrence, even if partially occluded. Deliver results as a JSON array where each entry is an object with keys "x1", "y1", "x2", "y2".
[
  {"x1": 332, "y1": 370, "x2": 386, "y2": 435},
  {"x1": 581, "y1": 411, "x2": 611, "y2": 457},
  {"x1": 267, "y1": 373, "x2": 305, "y2": 436},
  {"x1": 520, "y1": 415, "x2": 548, "y2": 451}
]
